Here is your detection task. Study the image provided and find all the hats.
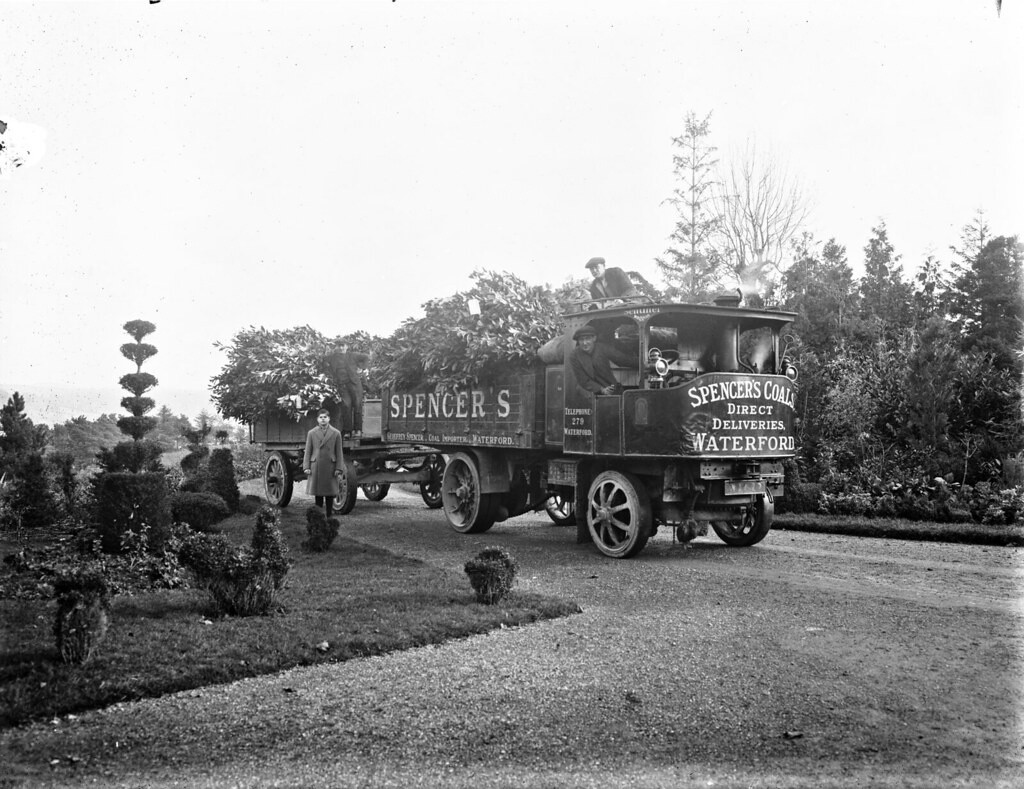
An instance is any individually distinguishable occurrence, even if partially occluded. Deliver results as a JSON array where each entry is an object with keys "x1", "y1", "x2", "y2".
[
  {"x1": 335, "y1": 337, "x2": 350, "y2": 343},
  {"x1": 585, "y1": 257, "x2": 605, "y2": 268},
  {"x1": 572, "y1": 327, "x2": 597, "y2": 341}
]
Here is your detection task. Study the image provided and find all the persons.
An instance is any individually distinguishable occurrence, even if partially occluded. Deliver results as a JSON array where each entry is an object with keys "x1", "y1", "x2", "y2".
[
  {"x1": 304, "y1": 408, "x2": 344, "y2": 516},
  {"x1": 586, "y1": 256, "x2": 638, "y2": 308},
  {"x1": 569, "y1": 325, "x2": 635, "y2": 396},
  {"x1": 326, "y1": 337, "x2": 370, "y2": 437}
]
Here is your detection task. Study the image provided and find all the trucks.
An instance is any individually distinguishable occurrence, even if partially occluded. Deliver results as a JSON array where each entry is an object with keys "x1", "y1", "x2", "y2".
[{"x1": 249, "y1": 287, "x2": 799, "y2": 559}]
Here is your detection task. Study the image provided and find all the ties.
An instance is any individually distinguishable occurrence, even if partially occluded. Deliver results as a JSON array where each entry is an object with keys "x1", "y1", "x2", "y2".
[{"x1": 599, "y1": 281, "x2": 609, "y2": 298}]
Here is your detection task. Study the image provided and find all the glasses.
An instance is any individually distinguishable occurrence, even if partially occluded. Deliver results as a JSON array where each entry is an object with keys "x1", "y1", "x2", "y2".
[{"x1": 337, "y1": 344, "x2": 348, "y2": 347}]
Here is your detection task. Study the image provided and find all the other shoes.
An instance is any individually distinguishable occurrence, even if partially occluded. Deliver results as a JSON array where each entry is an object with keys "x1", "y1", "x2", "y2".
[
  {"x1": 352, "y1": 430, "x2": 363, "y2": 439},
  {"x1": 343, "y1": 431, "x2": 351, "y2": 439}
]
